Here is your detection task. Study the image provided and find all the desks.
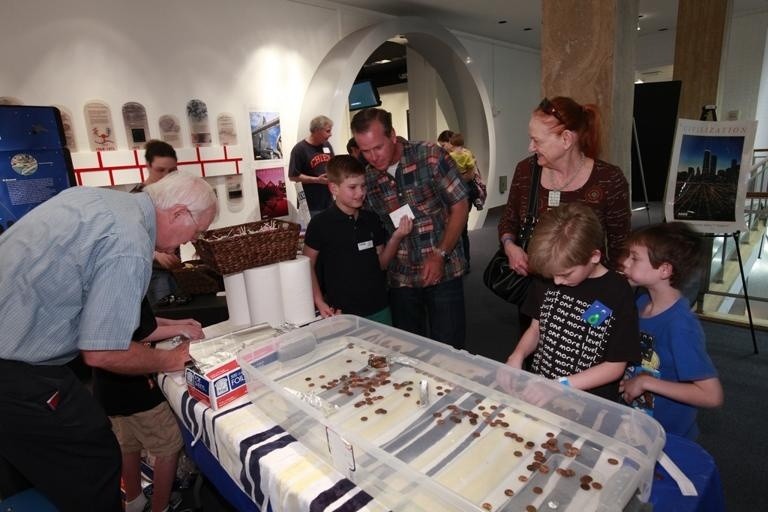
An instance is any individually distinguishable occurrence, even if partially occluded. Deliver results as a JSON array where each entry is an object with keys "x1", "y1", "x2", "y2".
[{"x1": 153, "y1": 313, "x2": 725, "y2": 512}]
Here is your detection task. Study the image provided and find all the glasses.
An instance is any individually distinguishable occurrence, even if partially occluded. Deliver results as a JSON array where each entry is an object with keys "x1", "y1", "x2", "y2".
[
  {"x1": 186, "y1": 209, "x2": 205, "y2": 241},
  {"x1": 540, "y1": 98, "x2": 568, "y2": 127}
]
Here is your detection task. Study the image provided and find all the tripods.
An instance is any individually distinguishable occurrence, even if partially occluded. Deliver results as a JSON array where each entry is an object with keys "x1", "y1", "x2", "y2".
[{"x1": 633, "y1": 104, "x2": 758, "y2": 354}]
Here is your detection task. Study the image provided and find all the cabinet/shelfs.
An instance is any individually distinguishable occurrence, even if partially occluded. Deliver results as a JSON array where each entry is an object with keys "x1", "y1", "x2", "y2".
[{"x1": 404, "y1": 31, "x2": 540, "y2": 208}]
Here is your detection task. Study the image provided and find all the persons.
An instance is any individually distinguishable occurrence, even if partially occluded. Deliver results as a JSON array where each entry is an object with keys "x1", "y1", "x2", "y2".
[
  {"x1": 83, "y1": 278, "x2": 204, "y2": 512},
  {"x1": 496, "y1": 94, "x2": 632, "y2": 348},
  {"x1": 346, "y1": 136, "x2": 369, "y2": 168},
  {"x1": 129, "y1": 139, "x2": 199, "y2": 317},
  {"x1": 288, "y1": 114, "x2": 336, "y2": 219},
  {"x1": 304, "y1": 153, "x2": 413, "y2": 330},
  {"x1": 498, "y1": 205, "x2": 643, "y2": 403},
  {"x1": 616, "y1": 220, "x2": 723, "y2": 443},
  {"x1": 350, "y1": 111, "x2": 474, "y2": 347},
  {"x1": 437, "y1": 130, "x2": 476, "y2": 272},
  {"x1": 0, "y1": 169, "x2": 219, "y2": 512}
]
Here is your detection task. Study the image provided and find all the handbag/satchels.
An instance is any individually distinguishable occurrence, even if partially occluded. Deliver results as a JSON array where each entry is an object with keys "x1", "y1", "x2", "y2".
[
  {"x1": 172, "y1": 260, "x2": 224, "y2": 294},
  {"x1": 483, "y1": 239, "x2": 533, "y2": 304},
  {"x1": 473, "y1": 173, "x2": 487, "y2": 204}
]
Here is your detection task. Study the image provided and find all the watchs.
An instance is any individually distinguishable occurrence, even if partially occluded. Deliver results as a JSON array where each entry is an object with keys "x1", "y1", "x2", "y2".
[{"x1": 554, "y1": 370, "x2": 571, "y2": 393}]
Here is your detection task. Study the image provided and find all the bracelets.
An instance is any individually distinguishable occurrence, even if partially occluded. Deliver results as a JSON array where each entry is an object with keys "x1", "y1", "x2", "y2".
[
  {"x1": 501, "y1": 233, "x2": 514, "y2": 250},
  {"x1": 434, "y1": 242, "x2": 448, "y2": 260}
]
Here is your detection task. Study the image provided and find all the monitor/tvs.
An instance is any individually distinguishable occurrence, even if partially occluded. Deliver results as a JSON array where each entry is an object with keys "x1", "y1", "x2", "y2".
[{"x1": 349, "y1": 79, "x2": 383, "y2": 112}]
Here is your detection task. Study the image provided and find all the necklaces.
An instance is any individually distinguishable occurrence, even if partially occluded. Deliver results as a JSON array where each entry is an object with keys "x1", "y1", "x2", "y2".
[{"x1": 542, "y1": 150, "x2": 591, "y2": 214}]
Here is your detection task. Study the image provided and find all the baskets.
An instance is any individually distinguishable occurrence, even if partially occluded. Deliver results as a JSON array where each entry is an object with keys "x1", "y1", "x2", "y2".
[{"x1": 192, "y1": 219, "x2": 301, "y2": 274}]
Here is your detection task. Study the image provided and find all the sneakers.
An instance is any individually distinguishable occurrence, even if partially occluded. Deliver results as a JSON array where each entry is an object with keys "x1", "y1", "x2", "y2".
[
  {"x1": 144, "y1": 489, "x2": 196, "y2": 512},
  {"x1": 475, "y1": 199, "x2": 484, "y2": 210}
]
